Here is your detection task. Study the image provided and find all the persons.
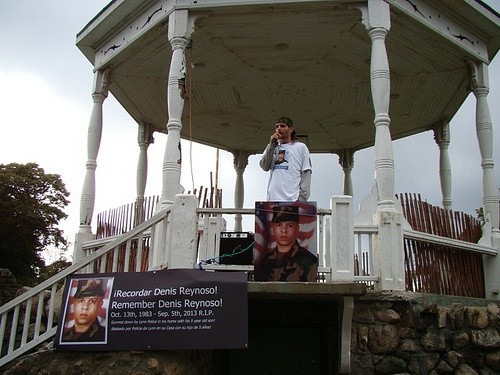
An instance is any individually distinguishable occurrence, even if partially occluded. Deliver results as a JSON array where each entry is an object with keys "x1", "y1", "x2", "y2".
[
  {"x1": 274, "y1": 149, "x2": 287, "y2": 164},
  {"x1": 260, "y1": 117, "x2": 312, "y2": 202},
  {"x1": 64, "y1": 279, "x2": 107, "y2": 342},
  {"x1": 257, "y1": 205, "x2": 317, "y2": 282}
]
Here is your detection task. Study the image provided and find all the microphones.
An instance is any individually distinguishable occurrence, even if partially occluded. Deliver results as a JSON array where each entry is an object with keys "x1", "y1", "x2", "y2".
[{"x1": 272, "y1": 132, "x2": 282, "y2": 143}]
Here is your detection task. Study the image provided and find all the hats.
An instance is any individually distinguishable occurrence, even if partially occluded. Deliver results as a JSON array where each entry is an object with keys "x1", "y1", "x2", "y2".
[
  {"x1": 73, "y1": 280, "x2": 105, "y2": 297},
  {"x1": 279, "y1": 150, "x2": 285, "y2": 154},
  {"x1": 276, "y1": 117, "x2": 294, "y2": 127},
  {"x1": 270, "y1": 206, "x2": 298, "y2": 222}
]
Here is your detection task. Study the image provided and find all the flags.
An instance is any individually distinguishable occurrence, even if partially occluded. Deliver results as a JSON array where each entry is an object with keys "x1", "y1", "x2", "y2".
[
  {"x1": 64, "y1": 280, "x2": 109, "y2": 332},
  {"x1": 254, "y1": 201, "x2": 315, "y2": 266}
]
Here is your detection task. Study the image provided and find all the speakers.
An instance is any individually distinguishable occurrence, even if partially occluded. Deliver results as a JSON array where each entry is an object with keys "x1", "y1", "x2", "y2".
[{"x1": 219, "y1": 231, "x2": 255, "y2": 266}]
